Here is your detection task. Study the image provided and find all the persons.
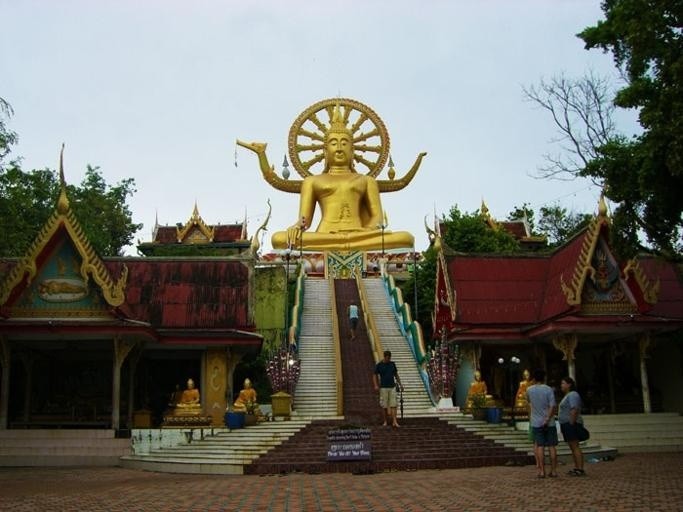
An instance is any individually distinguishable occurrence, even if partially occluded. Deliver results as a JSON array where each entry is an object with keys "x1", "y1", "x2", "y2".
[
  {"x1": 271, "y1": 118, "x2": 417, "y2": 250},
  {"x1": 557, "y1": 376, "x2": 585, "y2": 476},
  {"x1": 464, "y1": 369, "x2": 497, "y2": 409},
  {"x1": 371, "y1": 350, "x2": 404, "y2": 426},
  {"x1": 348, "y1": 302, "x2": 359, "y2": 339},
  {"x1": 231, "y1": 378, "x2": 261, "y2": 414},
  {"x1": 525, "y1": 371, "x2": 557, "y2": 478},
  {"x1": 514, "y1": 369, "x2": 533, "y2": 407},
  {"x1": 524, "y1": 403, "x2": 540, "y2": 468},
  {"x1": 171, "y1": 377, "x2": 202, "y2": 410}
]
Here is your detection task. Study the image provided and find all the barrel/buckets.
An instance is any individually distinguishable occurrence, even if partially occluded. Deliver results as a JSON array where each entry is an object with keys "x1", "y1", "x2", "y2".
[
  {"x1": 131, "y1": 426, "x2": 229, "y2": 456},
  {"x1": 485, "y1": 406, "x2": 504, "y2": 422}
]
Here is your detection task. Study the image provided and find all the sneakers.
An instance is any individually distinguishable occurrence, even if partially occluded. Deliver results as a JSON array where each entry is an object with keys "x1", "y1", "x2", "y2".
[
  {"x1": 534, "y1": 464, "x2": 557, "y2": 478},
  {"x1": 567, "y1": 467, "x2": 585, "y2": 476}
]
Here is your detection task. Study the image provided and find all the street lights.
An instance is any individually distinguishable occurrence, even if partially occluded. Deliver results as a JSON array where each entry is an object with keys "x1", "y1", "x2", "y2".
[{"x1": 498, "y1": 356, "x2": 520, "y2": 427}]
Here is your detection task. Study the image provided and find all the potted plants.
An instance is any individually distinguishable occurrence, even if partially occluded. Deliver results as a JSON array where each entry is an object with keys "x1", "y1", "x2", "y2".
[
  {"x1": 243, "y1": 401, "x2": 259, "y2": 426},
  {"x1": 467, "y1": 391, "x2": 490, "y2": 421}
]
